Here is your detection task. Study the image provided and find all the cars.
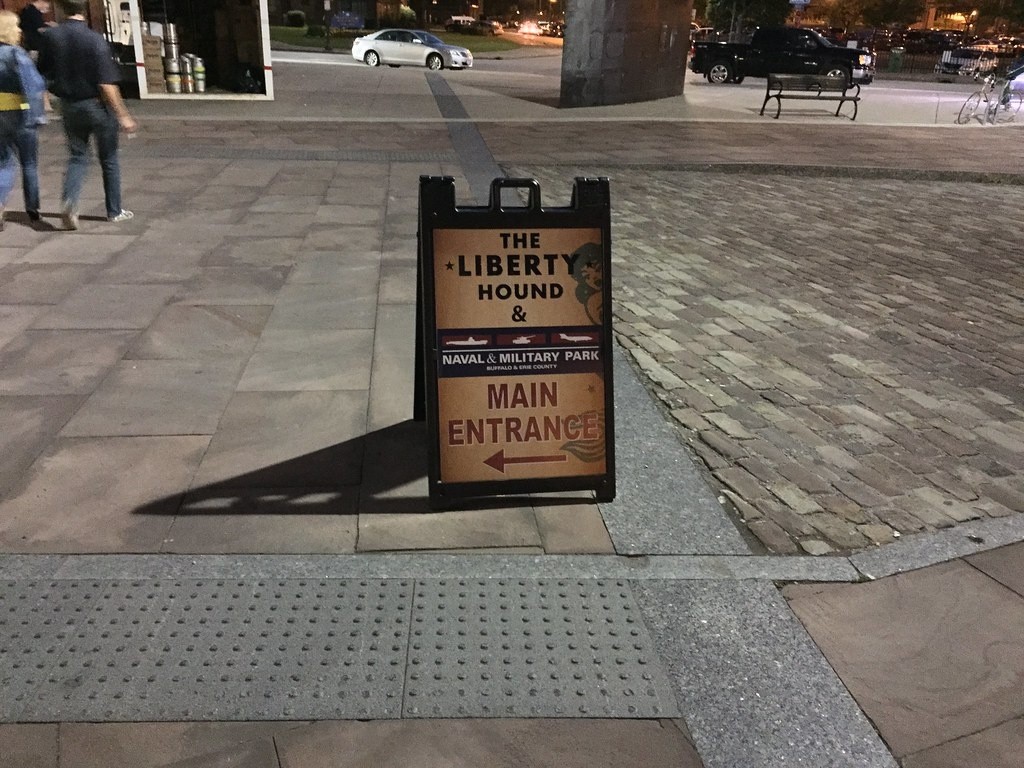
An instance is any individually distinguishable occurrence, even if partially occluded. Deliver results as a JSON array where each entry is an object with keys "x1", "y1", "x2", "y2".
[
  {"x1": 812, "y1": 26, "x2": 1024, "y2": 58},
  {"x1": 444, "y1": 16, "x2": 564, "y2": 37},
  {"x1": 933, "y1": 50, "x2": 999, "y2": 77},
  {"x1": 351, "y1": 28, "x2": 473, "y2": 71}
]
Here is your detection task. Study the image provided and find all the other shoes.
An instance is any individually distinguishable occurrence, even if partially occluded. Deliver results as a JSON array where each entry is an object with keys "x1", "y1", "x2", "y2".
[
  {"x1": 44, "y1": 110, "x2": 62, "y2": 120},
  {"x1": 0, "y1": 211, "x2": 5, "y2": 232},
  {"x1": 26, "y1": 207, "x2": 42, "y2": 222}
]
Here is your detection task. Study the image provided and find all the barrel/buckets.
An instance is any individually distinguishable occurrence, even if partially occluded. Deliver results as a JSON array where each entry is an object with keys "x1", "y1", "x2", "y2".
[
  {"x1": 163, "y1": 23, "x2": 181, "y2": 58},
  {"x1": 165, "y1": 53, "x2": 206, "y2": 93}
]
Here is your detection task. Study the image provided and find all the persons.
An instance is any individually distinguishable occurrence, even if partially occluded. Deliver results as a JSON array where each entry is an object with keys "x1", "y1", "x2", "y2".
[
  {"x1": 0, "y1": 9, "x2": 46, "y2": 231},
  {"x1": 38, "y1": 0, "x2": 135, "y2": 229},
  {"x1": 16, "y1": 0, "x2": 53, "y2": 113}
]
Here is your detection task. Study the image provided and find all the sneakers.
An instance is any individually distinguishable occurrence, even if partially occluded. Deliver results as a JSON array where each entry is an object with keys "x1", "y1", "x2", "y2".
[
  {"x1": 107, "y1": 209, "x2": 134, "y2": 223},
  {"x1": 62, "y1": 214, "x2": 79, "y2": 230}
]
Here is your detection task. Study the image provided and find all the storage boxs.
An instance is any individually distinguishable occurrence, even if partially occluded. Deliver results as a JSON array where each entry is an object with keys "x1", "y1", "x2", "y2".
[
  {"x1": 142, "y1": 35, "x2": 168, "y2": 96},
  {"x1": 148, "y1": 21, "x2": 165, "y2": 57},
  {"x1": 214, "y1": 0, "x2": 259, "y2": 68}
]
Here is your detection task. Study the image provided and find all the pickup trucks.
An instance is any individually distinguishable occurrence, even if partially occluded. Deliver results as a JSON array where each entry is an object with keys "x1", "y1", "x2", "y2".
[{"x1": 686, "y1": 24, "x2": 877, "y2": 88}]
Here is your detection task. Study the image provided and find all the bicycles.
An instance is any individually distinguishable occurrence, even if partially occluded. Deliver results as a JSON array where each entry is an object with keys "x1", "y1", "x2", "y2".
[{"x1": 955, "y1": 73, "x2": 1024, "y2": 126}]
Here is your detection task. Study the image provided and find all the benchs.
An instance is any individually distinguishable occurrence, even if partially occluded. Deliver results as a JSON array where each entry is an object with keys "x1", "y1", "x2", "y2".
[{"x1": 759, "y1": 73, "x2": 861, "y2": 121}]
